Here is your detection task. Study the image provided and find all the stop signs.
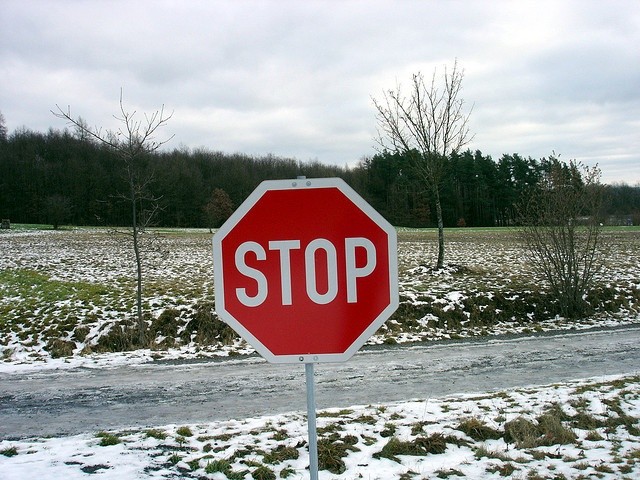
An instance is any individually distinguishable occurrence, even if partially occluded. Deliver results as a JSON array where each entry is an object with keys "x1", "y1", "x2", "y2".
[{"x1": 213, "y1": 178, "x2": 399, "y2": 363}]
